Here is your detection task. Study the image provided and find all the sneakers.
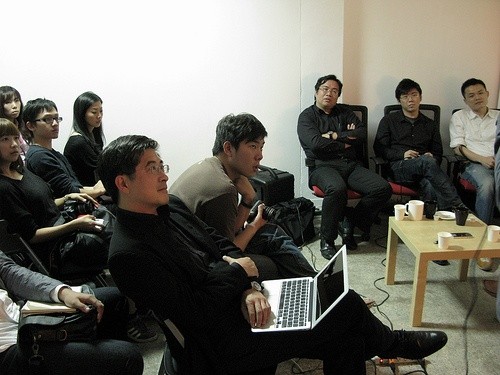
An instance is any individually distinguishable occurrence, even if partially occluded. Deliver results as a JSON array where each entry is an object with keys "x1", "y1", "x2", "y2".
[
  {"x1": 476, "y1": 257, "x2": 494, "y2": 272},
  {"x1": 127, "y1": 318, "x2": 158, "y2": 342}
]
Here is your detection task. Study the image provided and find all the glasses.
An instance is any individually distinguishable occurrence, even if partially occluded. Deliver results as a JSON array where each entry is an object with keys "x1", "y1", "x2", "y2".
[
  {"x1": 135, "y1": 164, "x2": 169, "y2": 175},
  {"x1": 32, "y1": 116, "x2": 62, "y2": 124},
  {"x1": 318, "y1": 87, "x2": 339, "y2": 95}
]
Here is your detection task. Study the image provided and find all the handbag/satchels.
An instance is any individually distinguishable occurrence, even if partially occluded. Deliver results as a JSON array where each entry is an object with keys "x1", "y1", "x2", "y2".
[
  {"x1": 18, "y1": 300, "x2": 100, "y2": 354},
  {"x1": 60, "y1": 229, "x2": 107, "y2": 274},
  {"x1": 271, "y1": 197, "x2": 316, "y2": 247}
]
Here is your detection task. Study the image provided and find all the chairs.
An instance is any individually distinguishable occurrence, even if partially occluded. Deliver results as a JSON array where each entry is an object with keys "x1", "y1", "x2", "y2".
[
  {"x1": 303, "y1": 103, "x2": 500, "y2": 243},
  {"x1": 148, "y1": 310, "x2": 189, "y2": 375},
  {"x1": 0, "y1": 218, "x2": 112, "y2": 288}
]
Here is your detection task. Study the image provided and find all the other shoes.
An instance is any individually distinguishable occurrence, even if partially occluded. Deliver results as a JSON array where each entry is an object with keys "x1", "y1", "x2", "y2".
[
  {"x1": 432, "y1": 259, "x2": 451, "y2": 266},
  {"x1": 459, "y1": 203, "x2": 473, "y2": 213}
]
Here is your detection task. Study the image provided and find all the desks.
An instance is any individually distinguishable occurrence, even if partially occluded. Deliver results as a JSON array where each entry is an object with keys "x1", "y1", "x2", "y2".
[{"x1": 384, "y1": 213, "x2": 500, "y2": 327}]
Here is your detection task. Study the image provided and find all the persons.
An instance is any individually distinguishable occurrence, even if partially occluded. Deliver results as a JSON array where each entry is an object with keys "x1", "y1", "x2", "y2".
[
  {"x1": 296, "y1": 75, "x2": 394, "y2": 261},
  {"x1": 0, "y1": 84, "x2": 159, "y2": 374},
  {"x1": 372, "y1": 78, "x2": 487, "y2": 265},
  {"x1": 98, "y1": 135, "x2": 448, "y2": 375},
  {"x1": 449, "y1": 78, "x2": 500, "y2": 272},
  {"x1": 166, "y1": 114, "x2": 376, "y2": 310}
]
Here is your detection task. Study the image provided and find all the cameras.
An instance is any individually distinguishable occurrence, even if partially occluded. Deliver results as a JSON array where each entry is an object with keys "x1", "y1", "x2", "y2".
[
  {"x1": 62, "y1": 198, "x2": 94, "y2": 224},
  {"x1": 246, "y1": 200, "x2": 276, "y2": 224}
]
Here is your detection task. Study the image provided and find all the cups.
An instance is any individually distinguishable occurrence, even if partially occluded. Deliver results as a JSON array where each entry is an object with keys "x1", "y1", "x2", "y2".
[
  {"x1": 405, "y1": 200, "x2": 424, "y2": 221},
  {"x1": 393, "y1": 204, "x2": 405, "y2": 221},
  {"x1": 454, "y1": 207, "x2": 469, "y2": 226},
  {"x1": 438, "y1": 232, "x2": 452, "y2": 249},
  {"x1": 487, "y1": 225, "x2": 500, "y2": 242},
  {"x1": 424, "y1": 200, "x2": 438, "y2": 219}
]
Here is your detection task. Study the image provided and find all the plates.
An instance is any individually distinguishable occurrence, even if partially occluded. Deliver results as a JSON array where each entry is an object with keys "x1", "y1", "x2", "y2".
[{"x1": 435, "y1": 211, "x2": 456, "y2": 219}]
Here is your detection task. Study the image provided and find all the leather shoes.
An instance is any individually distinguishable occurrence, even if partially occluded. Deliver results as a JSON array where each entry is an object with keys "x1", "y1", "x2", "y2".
[
  {"x1": 379, "y1": 330, "x2": 447, "y2": 358},
  {"x1": 337, "y1": 222, "x2": 358, "y2": 250},
  {"x1": 320, "y1": 239, "x2": 337, "y2": 260}
]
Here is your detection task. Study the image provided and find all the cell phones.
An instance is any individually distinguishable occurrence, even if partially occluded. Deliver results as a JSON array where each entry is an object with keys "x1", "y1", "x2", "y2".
[{"x1": 451, "y1": 233, "x2": 472, "y2": 238}]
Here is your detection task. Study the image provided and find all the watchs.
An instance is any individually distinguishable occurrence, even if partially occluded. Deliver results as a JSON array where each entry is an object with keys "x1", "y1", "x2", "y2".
[{"x1": 250, "y1": 280, "x2": 265, "y2": 294}]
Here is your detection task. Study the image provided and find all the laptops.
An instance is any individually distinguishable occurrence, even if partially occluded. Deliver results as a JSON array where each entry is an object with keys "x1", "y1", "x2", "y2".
[{"x1": 251, "y1": 244, "x2": 349, "y2": 333}]
